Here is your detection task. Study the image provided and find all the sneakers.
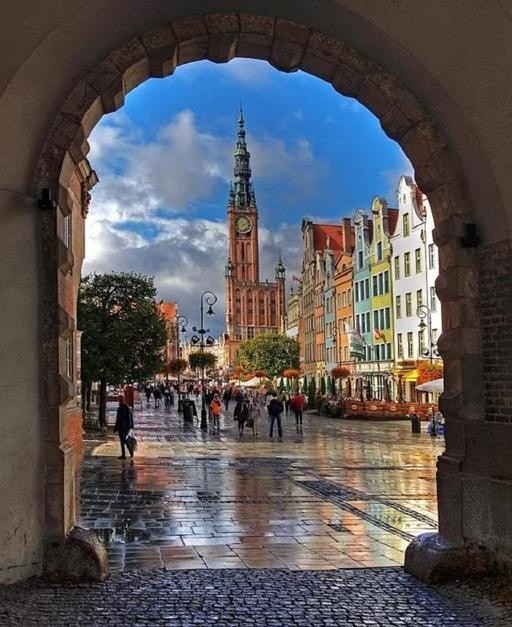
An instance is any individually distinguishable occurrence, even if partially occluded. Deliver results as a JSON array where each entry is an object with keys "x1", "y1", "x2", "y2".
[{"x1": 117, "y1": 455, "x2": 126, "y2": 460}]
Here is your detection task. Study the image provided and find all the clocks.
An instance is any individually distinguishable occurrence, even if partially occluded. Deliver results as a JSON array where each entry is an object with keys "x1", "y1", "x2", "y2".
[{"x1": 232, "y1": 214, "x2": 253, "y2": 234}]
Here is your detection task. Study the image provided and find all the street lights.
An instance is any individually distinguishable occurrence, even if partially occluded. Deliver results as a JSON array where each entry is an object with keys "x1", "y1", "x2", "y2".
[
  {"x1": 191, "y1": 289, "x2": 218, "y2": 429},
  {"x1": 329, "y1": 326, "x2": 343, "y2": 393},
  {"x1": 175, "y1": 315, "x2": 189, "y2": 412},
  {"x1": 416, "y1": 303, "x2": 442, "y2": 437}
]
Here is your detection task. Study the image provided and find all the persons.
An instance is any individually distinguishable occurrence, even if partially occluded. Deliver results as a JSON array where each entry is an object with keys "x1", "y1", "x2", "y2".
[
  {"x1": 143, "y1": 379, "x2": 305, "y2": 438},
  {"x1": 114, "y1": 398, "x2": 134, "y2": 459}
]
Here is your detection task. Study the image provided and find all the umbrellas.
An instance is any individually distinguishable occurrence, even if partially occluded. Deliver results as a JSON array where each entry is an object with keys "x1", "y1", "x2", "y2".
[{"x1": 415, "y1": 379, "x2": 445, "y2": 393}]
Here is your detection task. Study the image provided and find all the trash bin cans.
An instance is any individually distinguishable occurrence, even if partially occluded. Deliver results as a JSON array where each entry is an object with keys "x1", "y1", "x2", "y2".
[
  {"x1": 412, "y1": 414, "x2": 421, "y2": 433},
  {"x1": 183, "y1": 405, "x2": 194, "y2": 422}
]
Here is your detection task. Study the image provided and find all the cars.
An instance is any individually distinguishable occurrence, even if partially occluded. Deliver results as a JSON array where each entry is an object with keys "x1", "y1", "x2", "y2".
[{"x1": 106, "y1": 376, "x2": 156, "y2": 394}]
